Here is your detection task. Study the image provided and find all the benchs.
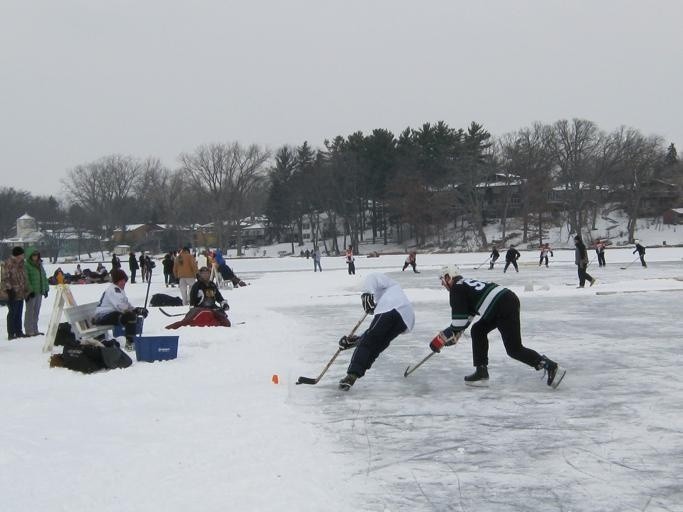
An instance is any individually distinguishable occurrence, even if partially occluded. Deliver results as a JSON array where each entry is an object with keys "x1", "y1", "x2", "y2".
[
  {"x1": 212, "y1": 263, "x2": 238, "y2": 288},
  {"x1": 64, "y1": 301, "x2": 116, "y2": 342}
]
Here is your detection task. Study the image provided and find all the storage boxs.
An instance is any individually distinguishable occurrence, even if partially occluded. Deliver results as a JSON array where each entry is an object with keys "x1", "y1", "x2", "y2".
[
  {"x1": 133, "y1": 336, "x2": 178, "y2": 363},
  {"x1": 116, "y1": 317, "x2": 143, "y2": 337}
]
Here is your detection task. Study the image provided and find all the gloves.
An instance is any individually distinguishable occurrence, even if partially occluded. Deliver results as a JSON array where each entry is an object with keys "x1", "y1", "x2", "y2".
[
  {"x1": 361, "y1": 293, "x2": 376, "y2": 315},
  {"x1": 135, "y1": 307, "x2": 148, "y2": 318},
  {"x1": 25, "y1": 292, "x2": 34, "y2": 301},
  {"x1": 220, "y1": 300, "x2": 229, "y2": 311},
  {"x1": 339, "y1": 336, "x2": 361, "y2": 350},
  {"x1": 45, "y1": 292, "x2": 47, "y2": 298},
  {"x1": 7, "y1": 288, "x2": 15, "y2": 300},
  {"x1": 429, "y1": 328, "x2": 465, "y2": 353}
]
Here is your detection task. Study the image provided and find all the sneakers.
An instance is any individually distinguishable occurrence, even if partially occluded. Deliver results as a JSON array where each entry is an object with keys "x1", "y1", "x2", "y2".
[
  {"x1": 8, "y1": 333, "x2": 44, "y2": 341},
  {"x1": 465, "y1": 365, "x2": 489, "y2": 380},
  {"x1": 339, "y1": 374, "x2": 357, "y2": 387},
  {"x1": 577, "y1": 279, "x2": 595, "y2": 289},
  {"x1": 540, "y1": 355, "x2": 557, "y2": 385}
]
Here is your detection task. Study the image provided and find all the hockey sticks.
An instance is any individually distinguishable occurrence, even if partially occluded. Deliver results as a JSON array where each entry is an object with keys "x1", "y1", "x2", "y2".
[
  {"x1": 404, "y1": 330, "x2": 466, "y2": 377},
  {"x1": 298, "y1": 312, "x2": 369, "y2": 385},
  {"x1": 620, "y1": 255, "x2": 642, "y2": 270},
  {"x1": 159, "y1": 308, "x2": 223, "y2": 317},
  {"x1": 474, "y1": 257, "x2": 491, "y2": 269}
]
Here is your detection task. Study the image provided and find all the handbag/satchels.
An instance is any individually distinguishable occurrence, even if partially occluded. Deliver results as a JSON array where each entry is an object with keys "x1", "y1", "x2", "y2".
[
  {"x1": 149, "y1": 294, "x2": 182, "y2": 307},
  {"x1": 52, "y1": 323, "x2": 132, "y2": 370}
]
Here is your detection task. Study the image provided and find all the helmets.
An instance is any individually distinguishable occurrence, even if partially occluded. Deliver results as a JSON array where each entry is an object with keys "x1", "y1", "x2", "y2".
[{"x1": 439, "y1": 264, "x2": 460, "y2": 279}]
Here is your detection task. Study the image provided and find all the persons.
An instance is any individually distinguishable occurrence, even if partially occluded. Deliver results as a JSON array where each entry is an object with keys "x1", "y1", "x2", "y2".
[
  {"x1": 633, "y1": 240, "x2": 647, "y2": 266},
  {"x1": 539, "y1": 243, "x2": 553, "y2": 267},
  {"x1": 430, "y1": 264, "x2": 558, "y2": 386},
  {"x1": 488, "y1": 246, "x2": 499, "y2": 270},
  {"x1": 595, "y1": 239, "x2": 607, "y2": 267},
  {"x1": 504, "y1": 244, "x2": 520, "y2": 273},
  {"x1": 338, "y1": 272, "x2": 415, "y2": 387},
  {"x1": 311, "y1": 245, "x2": 322, "y2": 272},
  {"x1": 573, "y1": 235, "x2": 596, "y2": 288},
  {"x1": 0, "y1": 247, "x2": 254, "y2": 350},
  {"x1": 345, "y1": 245, "x2": 356, "y2": 275},
  {"x1": 402, "y1": 251, "x2": 420, "y2": 272}
]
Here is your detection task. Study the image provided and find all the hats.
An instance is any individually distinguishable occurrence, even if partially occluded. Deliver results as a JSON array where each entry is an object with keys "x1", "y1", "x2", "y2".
[
  {"x1": 12, "y1": 247, "x2": 25, "y2": 256},
  {"x1": 635, "y1": 240, "x2": 639, "y2": 244},
  {"x1": 112, "y1": 269, "x2": 128, "y2": 283},
  {"x1": 574, "y1": 236, "x2": 582, "y2": 240}
]
